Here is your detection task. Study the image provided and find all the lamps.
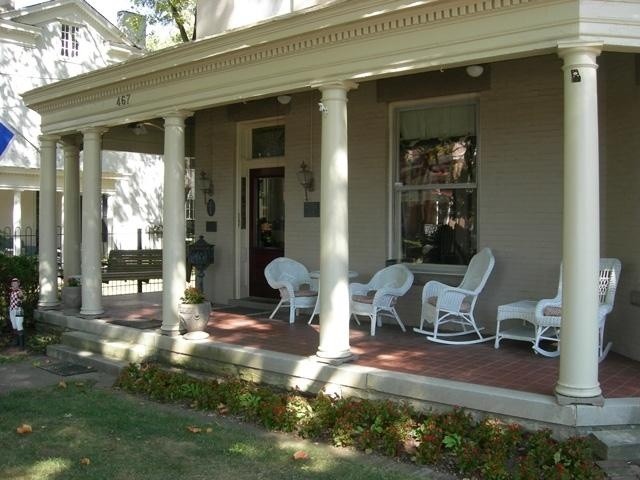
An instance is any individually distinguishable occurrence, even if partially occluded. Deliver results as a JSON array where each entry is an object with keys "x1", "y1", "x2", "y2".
[
  {"x1": 197, "y1": 170, "x2": 214, "y2": 197},
  {"x1": 466, "y1": 64, "x2": 485, "y2": 78},
  {"x1": 295, "y1": 160, "x2": 315, "y2": 194},
  {"x1": 277, "y1": 94, "x2": 293, "y2": 105}
]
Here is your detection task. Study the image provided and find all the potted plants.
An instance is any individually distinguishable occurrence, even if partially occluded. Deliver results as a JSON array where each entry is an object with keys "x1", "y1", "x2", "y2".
[
  {"x1": 60, "y1": 278, "x2": 82, "y2": 312},
  {"x1": 178, "y1": 284, "x2": 212, "y2": 339}
]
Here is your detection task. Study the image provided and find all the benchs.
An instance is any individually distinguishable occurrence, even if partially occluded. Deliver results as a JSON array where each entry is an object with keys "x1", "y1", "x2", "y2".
[{"x1": 101, "y1": 248, "x2": 162, "y2": 282}]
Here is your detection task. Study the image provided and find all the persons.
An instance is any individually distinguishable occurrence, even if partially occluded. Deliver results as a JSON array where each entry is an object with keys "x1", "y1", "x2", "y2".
[{"x1": 7, "y1": 278, "x2": 26, "y2": 353}]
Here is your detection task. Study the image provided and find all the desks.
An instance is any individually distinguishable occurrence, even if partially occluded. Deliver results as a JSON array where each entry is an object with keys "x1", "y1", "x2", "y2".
[{"x1": 494, "y1": 298, "x2": 560, "y2": 350}]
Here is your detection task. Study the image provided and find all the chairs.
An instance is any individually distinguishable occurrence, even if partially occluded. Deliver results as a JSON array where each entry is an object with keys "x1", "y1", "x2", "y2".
[
  {"x1": 349, "y1": 263, "x2": 415, "y2": 336},
  {"x1": 264, "y1": 256, "x2": 318, "y2": 325},
  {"x1": 412, "y1": 247, "x2": 496, "y2": 345},
  {"x1": 531, "y1": 257, "x2": 623, "y2": 365}
]
